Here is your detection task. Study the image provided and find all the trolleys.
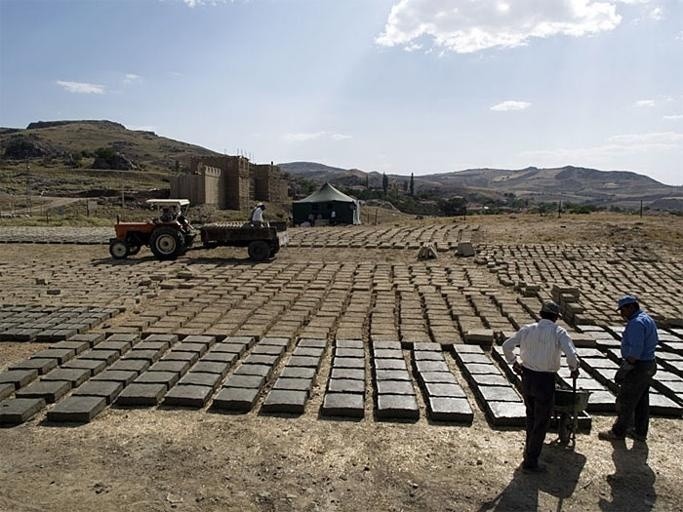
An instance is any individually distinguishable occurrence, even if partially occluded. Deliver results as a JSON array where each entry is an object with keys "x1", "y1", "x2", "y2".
[{"x1": 511, "y1": 367, "x2": 593, "y2": 445}]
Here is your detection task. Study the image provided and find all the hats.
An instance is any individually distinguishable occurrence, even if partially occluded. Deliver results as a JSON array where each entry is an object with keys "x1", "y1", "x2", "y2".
[
  {"x1": 543, "y1": 300, "x2": 562, "y2": 317},
  {"x1": 616, "y1": 295, "x2": 635, "y2": 312}
]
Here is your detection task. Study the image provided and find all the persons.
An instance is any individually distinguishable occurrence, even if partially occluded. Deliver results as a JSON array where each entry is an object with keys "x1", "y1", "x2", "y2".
[
  {"x1": 159, "y1": 201, "x2": 270, "y2": 234},
  {"x1": 501, "y1": 299, "x2": 581, "y2": 474},
  {"x1": 598, "y1": 294, "x2": 660, "y2": 442},
  {"x1": 304, "y1": 208, "x2": 336, "y2": 228}
]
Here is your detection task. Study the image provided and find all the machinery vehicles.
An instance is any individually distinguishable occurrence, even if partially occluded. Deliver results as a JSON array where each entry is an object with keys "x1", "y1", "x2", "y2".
[{"x1": 107, "y1": 196, "x2": 195, "y2": 263}]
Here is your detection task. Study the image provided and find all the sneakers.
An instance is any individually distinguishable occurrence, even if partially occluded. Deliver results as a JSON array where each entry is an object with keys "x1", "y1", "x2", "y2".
[
  {"x1": 627, "y1": 428, "x2": 647, "y2": 441},
  {"x1": 599, "y1": 432, "x2": 624, "y2": 440}
]
